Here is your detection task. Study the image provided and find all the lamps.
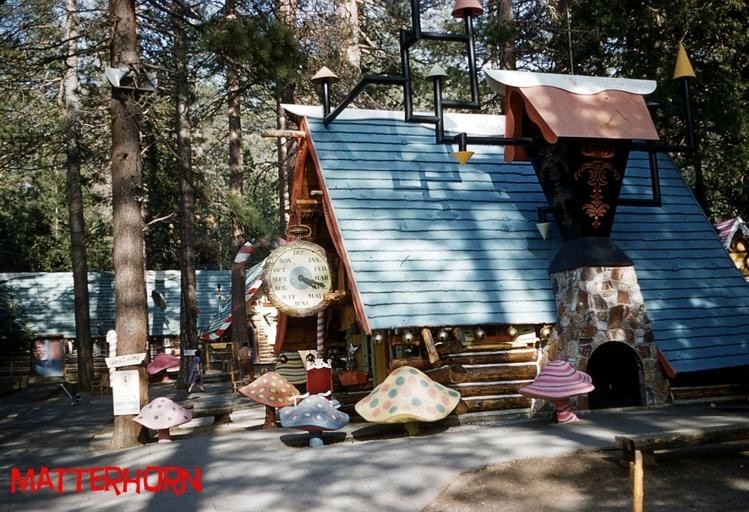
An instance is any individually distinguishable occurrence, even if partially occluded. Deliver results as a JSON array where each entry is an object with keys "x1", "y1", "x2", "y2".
[{"x1": 103, "y1": 64, "x2": 135, "y2": 90}]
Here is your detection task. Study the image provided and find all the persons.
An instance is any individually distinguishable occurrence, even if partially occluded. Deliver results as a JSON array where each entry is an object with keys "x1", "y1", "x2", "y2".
[
  {"x1": 186, "y1": 349, "x2": 206, "y2": 394},
  {"x1": 237, "y1": 342, "x2": 254, "y2": 380}
]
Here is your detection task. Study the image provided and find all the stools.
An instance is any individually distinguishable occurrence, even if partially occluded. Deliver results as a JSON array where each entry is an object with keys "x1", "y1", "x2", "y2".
[
  {"x1": 26, "y1": 377, "x2": 74, "y2": 413},
  {"x1": 233, "y1": 380, "x2": 249, "y2": 401},
  {"x1": 90, "y1": 375, "x2": 106, "y2": 398}
]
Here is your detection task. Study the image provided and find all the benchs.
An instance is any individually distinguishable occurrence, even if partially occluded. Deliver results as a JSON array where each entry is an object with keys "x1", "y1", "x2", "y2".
[
  {"x1": 614, "y1": 419, "x2": 749, "y2": 511},
  {"x1": 180, "y1": 405, "x2": 233, "y2": 425}
]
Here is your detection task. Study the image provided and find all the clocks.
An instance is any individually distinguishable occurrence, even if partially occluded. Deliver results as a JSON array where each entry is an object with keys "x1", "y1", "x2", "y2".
[{"x1": 262, "y1": 240, "x2": 336, "y2": 319}]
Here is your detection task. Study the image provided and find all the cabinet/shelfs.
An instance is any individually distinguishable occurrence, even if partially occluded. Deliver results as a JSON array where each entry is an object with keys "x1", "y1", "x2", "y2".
[{"x1": 202, "y1": 342, "x2": 234, "y2": 376}]
